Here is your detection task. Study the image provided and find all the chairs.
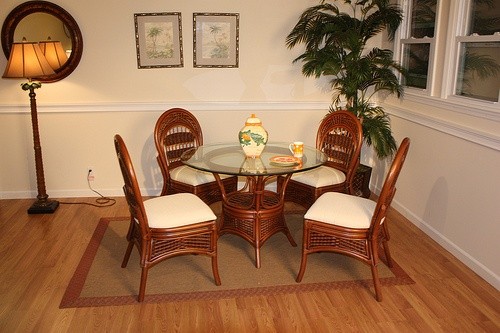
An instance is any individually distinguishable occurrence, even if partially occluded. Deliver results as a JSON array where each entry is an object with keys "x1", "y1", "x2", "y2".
[
  {"x1": 113, "y1": 134, "x2": 221, "y2": 302},
  {"x1": 295, "y1": 137, "x2": 412, "y2": 301},
  {"x1": 277, "y1": 110, "x2": 363, "y2": 210},
  {"x1": 154, "y1": 108, "x2": 238, "y2": 206}
]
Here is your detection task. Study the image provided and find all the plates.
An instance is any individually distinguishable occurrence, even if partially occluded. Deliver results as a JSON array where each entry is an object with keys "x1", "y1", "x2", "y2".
[{"x1": 269, "y1": 155, "x2": 302, "y2": 166}]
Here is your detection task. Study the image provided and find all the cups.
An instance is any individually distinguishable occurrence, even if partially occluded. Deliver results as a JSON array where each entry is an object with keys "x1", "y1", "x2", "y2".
[{"x1": 289, "y1": 142, "x2": 304, "y2": 158}]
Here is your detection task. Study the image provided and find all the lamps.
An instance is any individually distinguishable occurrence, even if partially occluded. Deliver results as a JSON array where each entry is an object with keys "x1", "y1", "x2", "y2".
[
  {"x1": 39, "y1": 37, "x2": 68, "y2": 70},
  {"x1": 2, "y1": 37, "x2": 60, "y2": 214}
]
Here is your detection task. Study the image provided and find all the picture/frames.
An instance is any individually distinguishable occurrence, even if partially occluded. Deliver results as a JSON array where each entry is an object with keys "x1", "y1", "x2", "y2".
[
  {"x1": 134, "y1": 12, "x2": 184, "y2": 69},
  {"x1": 193, "y1": 12, "x2": 239, "y2": 68}
]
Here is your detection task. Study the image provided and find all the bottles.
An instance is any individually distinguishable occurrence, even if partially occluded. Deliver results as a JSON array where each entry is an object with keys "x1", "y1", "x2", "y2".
[{"x1": 239, "y1": 114, "x2": 268, "y2": 158}]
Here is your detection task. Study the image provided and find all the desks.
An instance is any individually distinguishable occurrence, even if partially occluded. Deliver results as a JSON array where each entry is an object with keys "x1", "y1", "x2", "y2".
[{"x1": 181, "y1": 142, "x2": 329, "y2": 268}]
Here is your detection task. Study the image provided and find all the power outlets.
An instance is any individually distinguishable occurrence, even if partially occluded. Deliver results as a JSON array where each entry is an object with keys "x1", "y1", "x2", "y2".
[{"x1": 88, "y1": 166, "x2": 95, "y2": 176}]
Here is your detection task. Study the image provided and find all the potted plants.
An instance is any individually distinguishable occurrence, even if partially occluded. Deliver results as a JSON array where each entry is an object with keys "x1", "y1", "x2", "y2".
[{"x1": 285, "y1": 0, "x2": 410, "y2": 198}]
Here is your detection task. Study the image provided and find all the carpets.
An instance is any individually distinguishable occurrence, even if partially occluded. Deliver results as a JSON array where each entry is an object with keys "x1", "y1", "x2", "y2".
[{"x1": 58, "y1": 212, "x2": 417, "y2": 309}]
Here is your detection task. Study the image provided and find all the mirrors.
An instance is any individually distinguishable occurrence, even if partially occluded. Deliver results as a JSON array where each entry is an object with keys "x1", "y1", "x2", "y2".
[{"x1": 0, "y1": 0, "x2": 84, "y2": 83}]
